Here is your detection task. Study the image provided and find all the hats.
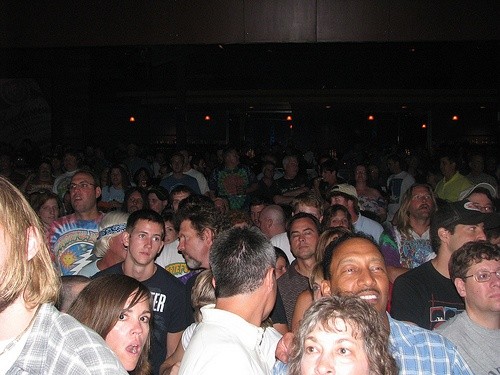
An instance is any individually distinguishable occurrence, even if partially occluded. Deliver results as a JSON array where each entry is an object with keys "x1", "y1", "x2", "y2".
[
  {"x1": 458, "y1": 182, "x2": 497, "y2": 201},
  {"x1": 330, "y1": 183, "x2": 364, "y2": 211}
]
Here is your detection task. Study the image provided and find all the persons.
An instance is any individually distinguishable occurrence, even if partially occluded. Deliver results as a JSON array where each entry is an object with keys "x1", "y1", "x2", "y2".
[
  {"x1": 0, "y1": 143, "x2": 500, "y2": 375},
  {"x1": 91, "y1": 207, "x2": 195, "y2": 375},
  {"x1": 432, "y1": 239, "x2": 500, "y2": 375},
  {"x1": 273, "y1": 232, "x2": 473, "y2": 375},
  {"x1": 175, "y1": 224, "x2": 295, "y2": 375},
  {"x1": 39, "y1": 170, "x2": 105, "y2": 276},
  {"x1": 66, "y1": 273, "x2": 152, "y2": 375},
  {"x1": 390, "y1": 201, "x2": 486, "y2": 330},
  {"x1": 287, "y1": 294, "x2": 400, "y2": 375},
  {"x1": 0, "y1": 177, "x2": 130, "y2": 375}
]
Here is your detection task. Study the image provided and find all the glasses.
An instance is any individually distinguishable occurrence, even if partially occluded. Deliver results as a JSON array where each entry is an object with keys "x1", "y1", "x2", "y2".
[
  {"x1": 464, "y1": 269, "x2": 500, "y2": 283},
  {"x1": 475, "y1": 205, "x2": 497, "y2": 212},
  {"x1": 68, "y1": 180, "x2": 97, "y2": 191}
]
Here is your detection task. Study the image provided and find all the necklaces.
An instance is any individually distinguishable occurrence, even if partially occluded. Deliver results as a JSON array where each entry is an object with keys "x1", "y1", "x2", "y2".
[{"x1": 0, "y1": 302, "x2": 43, "y2": 358}]
